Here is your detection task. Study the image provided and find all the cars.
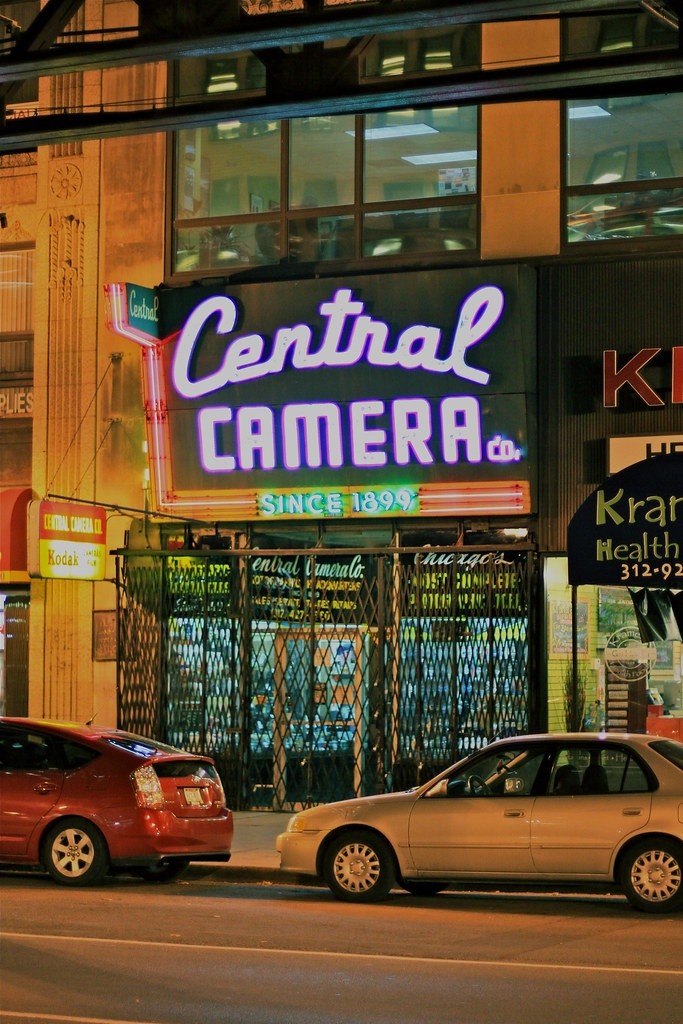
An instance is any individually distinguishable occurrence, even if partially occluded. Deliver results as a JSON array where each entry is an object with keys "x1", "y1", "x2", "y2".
[
  {"x1": 1, "y1": 713, "x2": 231, "y2": 889},
  {"x1": 273, "y1": 730, "x2": 683, "y2": 913}
]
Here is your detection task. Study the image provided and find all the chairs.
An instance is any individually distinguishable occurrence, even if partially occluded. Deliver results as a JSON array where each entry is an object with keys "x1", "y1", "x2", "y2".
[{"x1": 553, "y1": 762, "x2": 606, "y2": 795}]
